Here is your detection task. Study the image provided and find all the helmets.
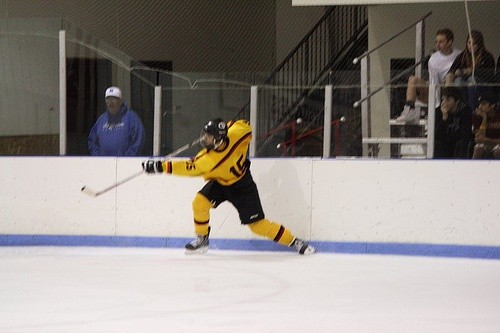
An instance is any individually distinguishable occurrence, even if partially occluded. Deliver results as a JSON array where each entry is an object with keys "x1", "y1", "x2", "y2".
[{"x1": 204, "y1": 118, "x2": 228, "y2": 140}]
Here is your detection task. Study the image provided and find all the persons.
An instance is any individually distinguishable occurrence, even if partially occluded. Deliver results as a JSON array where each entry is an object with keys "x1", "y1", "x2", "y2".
[
  {"x1": 396, "y1": 28, "x2": 500, "y2": 160},
  {"x1": 141, "y1": 117, "x2": 315, "y2": 256},
  {"x1": 88, "y1": 86, "x2": 146, "y2": 156}
]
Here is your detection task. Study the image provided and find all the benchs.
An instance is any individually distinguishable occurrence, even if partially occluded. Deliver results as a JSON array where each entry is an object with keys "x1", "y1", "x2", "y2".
[{"x1": 362, "y1": 102, "x2": 428, "y2": 158}]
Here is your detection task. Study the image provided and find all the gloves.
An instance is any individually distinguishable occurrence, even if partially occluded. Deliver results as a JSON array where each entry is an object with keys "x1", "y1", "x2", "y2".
[{"x1": 142, "y1": 160, "x2": 163, "y2": 173}]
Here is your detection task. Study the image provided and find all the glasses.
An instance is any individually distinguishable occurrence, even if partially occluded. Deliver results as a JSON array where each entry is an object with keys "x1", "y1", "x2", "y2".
[{"x1": 106, "y1": 98, "x2": 119, "y2": 102}]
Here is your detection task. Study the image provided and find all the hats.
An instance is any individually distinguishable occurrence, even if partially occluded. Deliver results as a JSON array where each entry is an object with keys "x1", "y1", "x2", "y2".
[
  {"x1": 105, "y1": 86, "x2": 122, "y2": 99},
  {"x1": 478, "y1": 90, "x2": 498, "y2": 102}
]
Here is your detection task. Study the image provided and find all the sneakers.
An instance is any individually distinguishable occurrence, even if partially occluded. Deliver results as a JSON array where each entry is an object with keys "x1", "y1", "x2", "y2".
[
  {"x1": 292, "y1": 239, "x2": 316, "y2": 256},
  {"x1": 185, "y1": 236, "x2": 209, "y2": 254},
  {"x1": 388, "y1": 104, "x2": 410, "y2": 125},
  {"x1": 397, "y1": 107, "x2": 420, "y2": 125}
]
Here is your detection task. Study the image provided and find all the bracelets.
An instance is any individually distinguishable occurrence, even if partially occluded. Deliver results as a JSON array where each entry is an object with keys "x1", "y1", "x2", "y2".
[{"x1": 480, "y1": 126, "x2": 486, "y2": 129}]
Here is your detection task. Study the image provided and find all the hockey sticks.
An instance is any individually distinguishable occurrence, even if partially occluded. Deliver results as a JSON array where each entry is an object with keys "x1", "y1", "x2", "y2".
[{"x1": 80, "y1": 136, "x2": 204, "y2": 198}]
